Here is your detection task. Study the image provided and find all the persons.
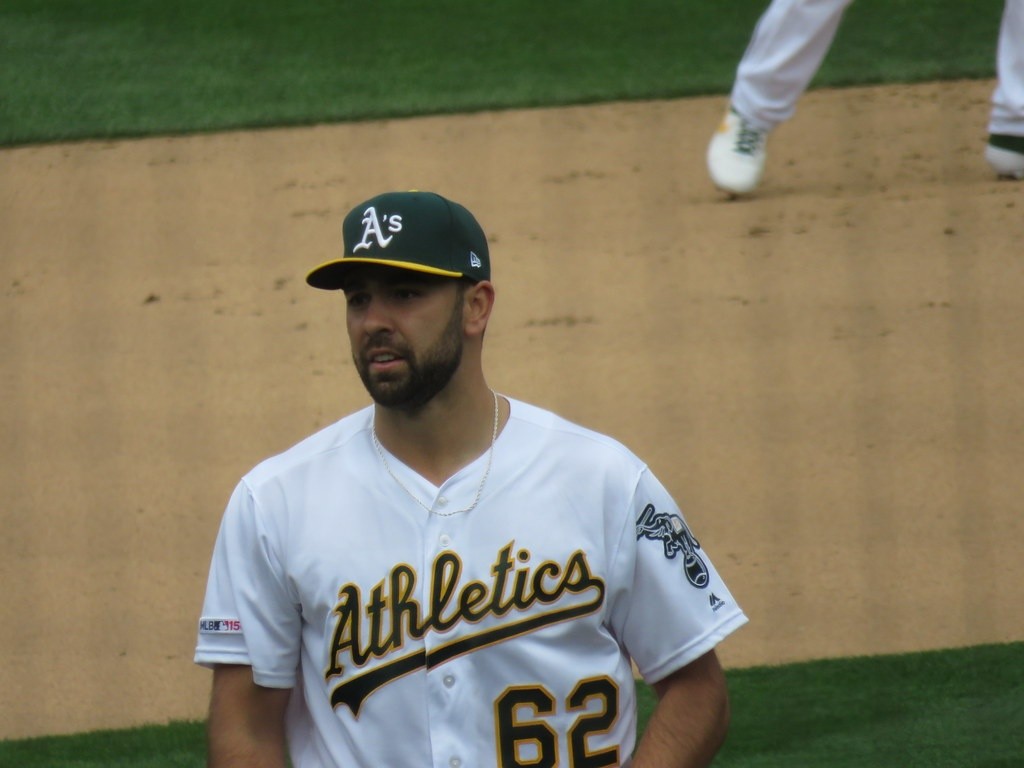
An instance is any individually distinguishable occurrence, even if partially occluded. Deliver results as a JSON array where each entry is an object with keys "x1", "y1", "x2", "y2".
[
  {"x1": 194, "y1": 187, "x2": 751, "y2": 767},
  {"x1": 705, "y1": 0, "x2": 1024, "y2": 194}
]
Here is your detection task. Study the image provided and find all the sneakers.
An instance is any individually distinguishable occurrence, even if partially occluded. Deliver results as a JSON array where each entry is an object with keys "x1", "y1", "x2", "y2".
[
  {"x1": 983, "y1": 131, "x2": 1020, "y2": 177},
  {"x1": 705, "y1": 108, "x2": 771, "y2": 198}
]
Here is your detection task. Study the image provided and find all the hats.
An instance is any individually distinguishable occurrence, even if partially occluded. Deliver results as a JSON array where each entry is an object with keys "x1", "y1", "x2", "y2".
[{"x1": 303, "y1": 187, "x2": 491, "y2": 290}]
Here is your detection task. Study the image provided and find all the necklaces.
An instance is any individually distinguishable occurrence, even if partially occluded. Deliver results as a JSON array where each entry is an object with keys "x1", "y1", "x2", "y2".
[{"x1": 370, "y1": 389, "x2": 499, "y2": 516}]
